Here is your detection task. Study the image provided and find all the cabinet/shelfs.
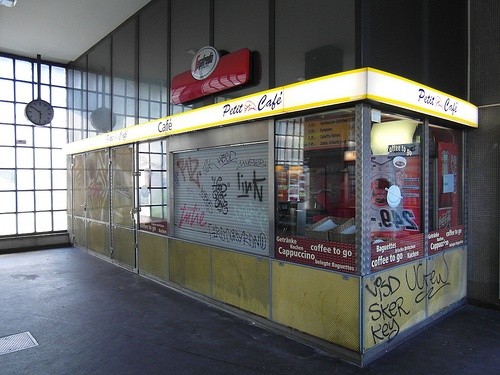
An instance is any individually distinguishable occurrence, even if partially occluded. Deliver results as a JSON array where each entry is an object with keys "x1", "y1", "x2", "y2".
[{"x1": 276, "y1": 165, "x2": 311, "y2": 233}]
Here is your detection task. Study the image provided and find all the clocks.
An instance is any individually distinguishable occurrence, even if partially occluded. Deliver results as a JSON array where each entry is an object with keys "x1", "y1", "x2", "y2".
[{"x1": 25, "y1": 99, "x2": 54, "y2": 126}]
